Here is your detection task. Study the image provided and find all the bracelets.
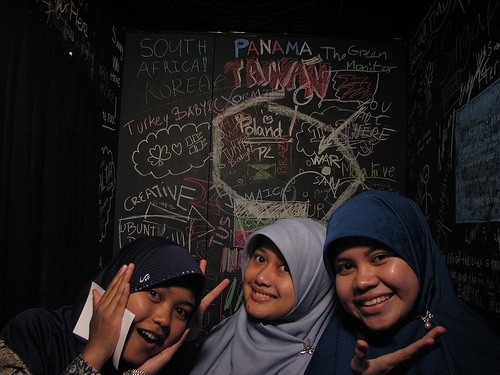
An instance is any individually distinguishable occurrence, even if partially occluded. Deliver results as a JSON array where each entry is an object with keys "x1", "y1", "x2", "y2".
[{"x1": 123, "y1": 369, "x2": 152, "y2": 375}]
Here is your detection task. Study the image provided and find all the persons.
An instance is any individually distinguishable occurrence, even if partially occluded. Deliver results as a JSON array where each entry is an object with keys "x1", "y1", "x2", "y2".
[
  {"x1": 186, "y1": 216, "x2": 340, "y2": 375},
  {"x1": 0, "y1": 235, "x2": 206, "y2": 375},
  {"x1": 304, "y1": 190, "x2": 500, "y2": 375}
]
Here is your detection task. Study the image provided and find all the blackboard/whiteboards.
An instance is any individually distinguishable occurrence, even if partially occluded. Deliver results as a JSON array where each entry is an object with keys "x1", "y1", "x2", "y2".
[
  {"x1": 116, "y1": 34, "x2": 412, "y2": 339},
  {"x1": 403, "y1": 0, "x2": 499, "y2": 375}
]
